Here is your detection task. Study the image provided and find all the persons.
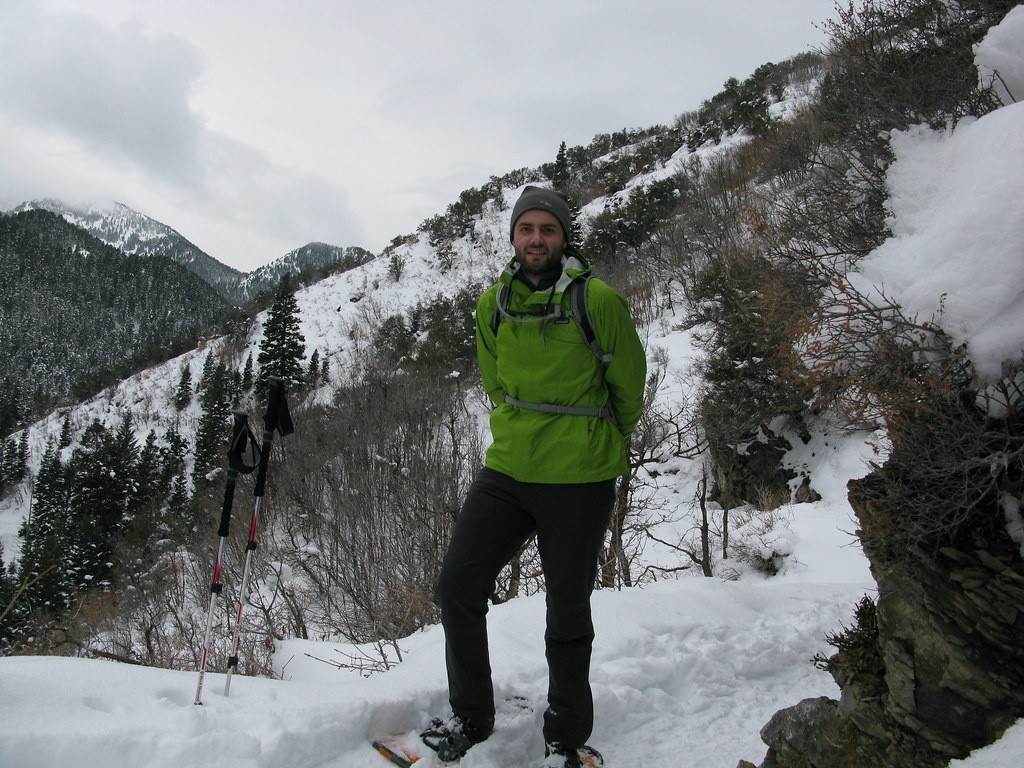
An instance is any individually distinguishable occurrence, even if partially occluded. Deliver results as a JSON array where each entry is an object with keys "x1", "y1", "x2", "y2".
[{"x1": 419, "y1": 186, "x2": 647, "y2": 768}]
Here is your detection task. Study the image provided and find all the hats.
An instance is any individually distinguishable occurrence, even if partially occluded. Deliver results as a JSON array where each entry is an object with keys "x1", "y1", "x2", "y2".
[{"x1": 509, "y1": 186, "x2": 571, "y2": 243}]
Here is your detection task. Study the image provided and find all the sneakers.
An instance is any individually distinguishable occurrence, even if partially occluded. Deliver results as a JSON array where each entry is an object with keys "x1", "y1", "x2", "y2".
[
  {"x1": 420, "y1": 714, "x2": 495, "y2": 763},
  {"x1": 545, "y1": 737, "x2": 603, "y2": 768}
]
